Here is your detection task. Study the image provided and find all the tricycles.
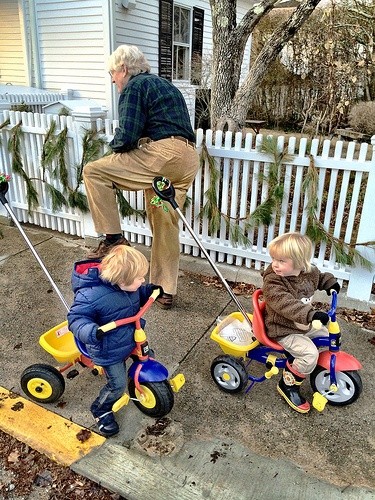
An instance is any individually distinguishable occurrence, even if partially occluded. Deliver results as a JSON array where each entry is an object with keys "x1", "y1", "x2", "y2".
[
  {"x1": 209, "y1": 289, "x2": 362, "y2": 412},
  {"x1": 20, "y1": 289, "x2": 186, "y2": 417}
]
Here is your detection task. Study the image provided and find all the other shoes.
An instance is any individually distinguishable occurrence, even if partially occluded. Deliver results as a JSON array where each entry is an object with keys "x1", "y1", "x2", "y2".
[
  {"x1": 155, "y1": 293, "x2": 173, "y2": 310},
  {"x1": 86, "y1": 236, "x2": 131, "y2": 259}
]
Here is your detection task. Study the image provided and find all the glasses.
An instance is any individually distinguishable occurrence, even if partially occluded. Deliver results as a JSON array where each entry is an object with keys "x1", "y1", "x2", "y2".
[{"x1": 108, "y1": 67, "x2": 122, "y2": 78}]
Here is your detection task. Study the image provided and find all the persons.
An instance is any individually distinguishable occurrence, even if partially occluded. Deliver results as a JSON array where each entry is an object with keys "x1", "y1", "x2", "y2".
[
  {"x1": 260, "y1": 231, "x2": 341, "y2": 414},
  {"x1": 66, "y1": 244, "x2": 165, "y2": 436},
  {"x1": 80, "y1": 43, "x2": 201, "y2": 310}
]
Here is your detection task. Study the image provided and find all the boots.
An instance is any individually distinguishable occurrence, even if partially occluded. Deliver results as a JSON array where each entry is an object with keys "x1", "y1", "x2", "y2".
[{"x1": 277, "y1": 359, "x2": 311, "y2": 414}]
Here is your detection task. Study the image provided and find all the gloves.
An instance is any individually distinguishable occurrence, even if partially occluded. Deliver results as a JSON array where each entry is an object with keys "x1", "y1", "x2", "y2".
[
  {"x1": 96, "y1": 329, "x2": 104, "y2": 340},
  {"x1": 326, "y1": 282, "x2": 340, "y2": 296},
  {"x1": 312, "y1": 312, "x2": 329, "y2": 325},
  {"x1": 151, "y1": 285, "x2": 163, "y2": 298}
]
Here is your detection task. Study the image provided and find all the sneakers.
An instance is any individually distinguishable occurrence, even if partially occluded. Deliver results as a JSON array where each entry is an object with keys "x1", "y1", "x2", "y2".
[{"x1": 90, "y1": 403, "x2": 120, "y2": 436}]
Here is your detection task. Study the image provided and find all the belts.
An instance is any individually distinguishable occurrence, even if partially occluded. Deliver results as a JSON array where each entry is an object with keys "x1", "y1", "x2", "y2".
[{"x1": 167, "y1": 136, "x2": 196, "y2": 148}]
